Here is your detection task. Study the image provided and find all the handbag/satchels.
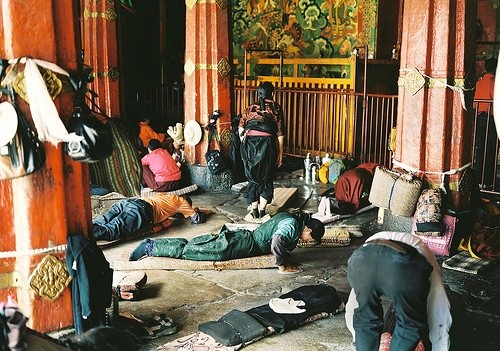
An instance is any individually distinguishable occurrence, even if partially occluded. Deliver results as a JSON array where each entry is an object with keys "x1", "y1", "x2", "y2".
[
  {"x1": 0, "y1": 59, "x2": 45, "y2": 180},
  {"x1": 66, "y1": 73, "x2": 143, "y2": 197},
  {"x1": 62, "y1": 67, "x2": 113, "y2": 163},
  {"x1": 457, "y1": 222, "x2": 500, "y2": 260},
  {"x1": 204, "y1": 127, "x2": 225, "y2": 174}
]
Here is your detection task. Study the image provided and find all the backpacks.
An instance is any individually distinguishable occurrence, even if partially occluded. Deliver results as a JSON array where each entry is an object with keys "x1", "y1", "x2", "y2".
[
  {"x1": 414, "y1": 188, "x2": 445, "y2": 237},
  {"x1": 329, "y1": 159, "x2": 346, "y2": 185}
]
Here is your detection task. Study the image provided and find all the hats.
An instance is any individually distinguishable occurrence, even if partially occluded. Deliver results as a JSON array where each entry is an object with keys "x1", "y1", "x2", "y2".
[{"x1": 184, "y1": 120, "x2": 202, "y2": 146}]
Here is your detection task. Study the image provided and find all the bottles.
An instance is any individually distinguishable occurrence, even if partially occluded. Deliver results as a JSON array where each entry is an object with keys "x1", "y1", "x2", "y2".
[
  {"x1": 303, "y1": 152, "x2": 332, "y2": 181},
  {"x1": 5, "y1": 295, "x2": 18, "y2": 315}
]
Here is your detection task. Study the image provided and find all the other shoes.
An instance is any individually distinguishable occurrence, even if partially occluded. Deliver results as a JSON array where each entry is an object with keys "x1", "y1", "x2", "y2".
[{"x1": 478, "y1": 183, "x2": 493, "y2": 189}]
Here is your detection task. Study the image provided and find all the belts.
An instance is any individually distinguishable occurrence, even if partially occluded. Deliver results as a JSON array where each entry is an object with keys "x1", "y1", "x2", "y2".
[{"x1": 365, "y1": 239, "x2": 408, "y2": 255}]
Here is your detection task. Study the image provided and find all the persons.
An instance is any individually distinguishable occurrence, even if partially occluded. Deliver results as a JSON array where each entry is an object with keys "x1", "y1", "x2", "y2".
[
  {"x1": 346, "y1": 230, "x2": 454, "y2": 351},
  {"x1": 92, "y1": 193, "x2": 214, "y2": 242},
  {"x1": 130, "y1": 210, "x2": 324, "y2": 274},
  {"x1": 138, "y1": 138, "x2": 189, "y2": 193},
  {"x1": 238, "y1": 81, "x2": 285, "y2": 219},
  {"x1": 137, "y1": 113, "x2": 174, "y2": 149},
  {"x1": 474, "y1": 57, "x2": 497, "y2": 196},
  {"x1": 317, "y1": 162, "x2": 382, "y2": 214}
]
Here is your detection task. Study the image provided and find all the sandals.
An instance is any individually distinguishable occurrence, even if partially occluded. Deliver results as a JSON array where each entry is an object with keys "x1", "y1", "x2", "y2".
[
  {"x1": 133, "y1": 313, "x2": 167, "y2": 333},
  {"x1": 140, "y1": 316, "x2": 178, "y2": 340}
]
